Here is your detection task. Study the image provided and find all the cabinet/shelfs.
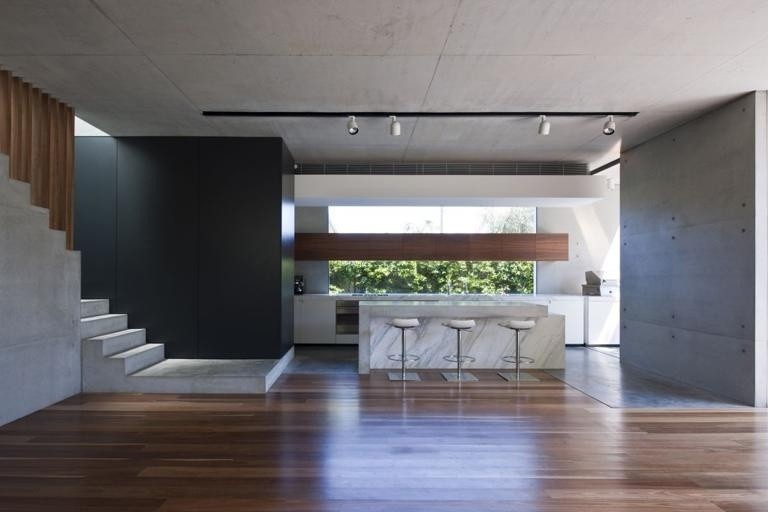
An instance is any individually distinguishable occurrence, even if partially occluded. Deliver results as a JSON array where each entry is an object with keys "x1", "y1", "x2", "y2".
[
  {"x1": 294, "y1": 232, "x2": 569, "y2": 261},
  {"x1": 294, "y1": 294, "x2": 332, "y2": 344},
  {"x1": 548, "y1": 294, "x2": 585, "y2": 346}
]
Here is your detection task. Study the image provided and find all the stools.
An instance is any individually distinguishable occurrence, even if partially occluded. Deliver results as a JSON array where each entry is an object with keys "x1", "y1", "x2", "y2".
[{"x1": 386, "y1": 317, "x2": 537, "y2": 384}]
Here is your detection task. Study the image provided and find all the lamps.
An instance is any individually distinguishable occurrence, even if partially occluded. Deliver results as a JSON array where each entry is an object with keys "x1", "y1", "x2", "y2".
[{"x1": 346, "y1": 114, "x2": 616, "y2": 136}]
